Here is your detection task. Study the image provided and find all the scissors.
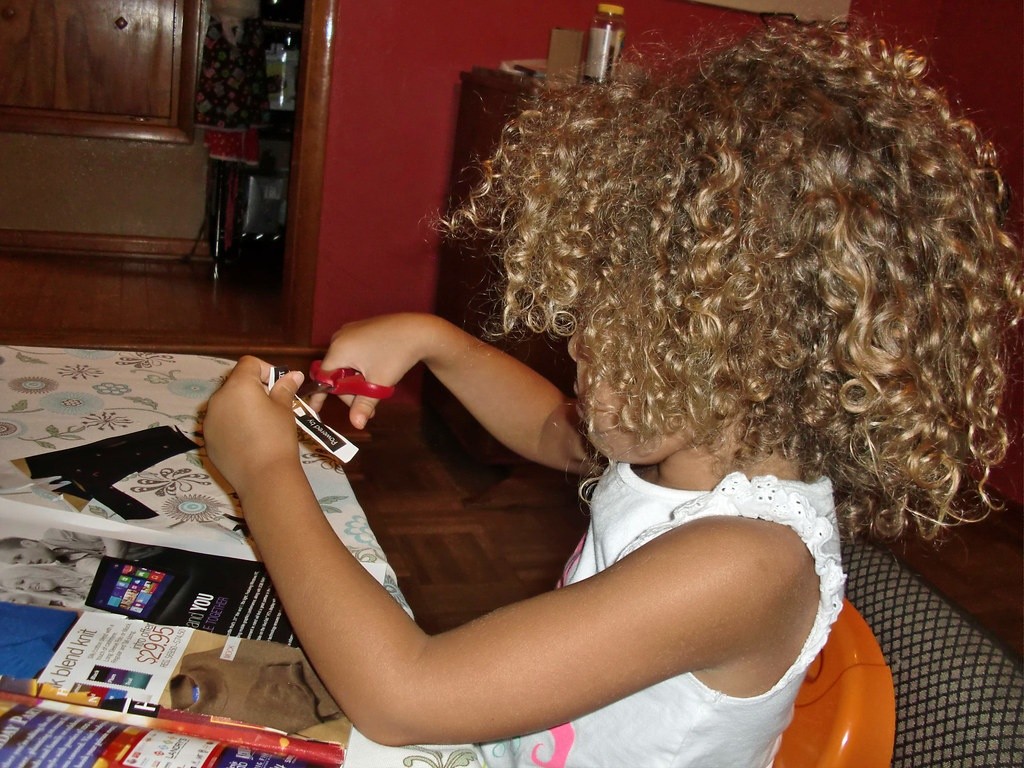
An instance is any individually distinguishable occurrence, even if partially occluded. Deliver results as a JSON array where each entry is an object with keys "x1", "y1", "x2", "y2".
[{"x1": 301, "y1": 361, "x2": 395, "y2": 409}]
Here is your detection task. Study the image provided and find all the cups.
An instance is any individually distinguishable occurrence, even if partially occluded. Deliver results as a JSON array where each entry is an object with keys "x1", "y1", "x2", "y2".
[{"x1": 266, "y1": 45, "x2": 299, "y2": 112}]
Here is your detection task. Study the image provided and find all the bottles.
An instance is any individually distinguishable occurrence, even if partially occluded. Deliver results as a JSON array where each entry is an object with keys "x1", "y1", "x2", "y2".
[{"x1": 584, "y1": 4, "x2": 627, "y2": 85}]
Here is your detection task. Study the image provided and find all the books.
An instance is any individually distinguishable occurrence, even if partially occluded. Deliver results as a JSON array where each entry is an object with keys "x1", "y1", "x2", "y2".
[{"x1": 0, "y1": 497, "x2": 388, "y2": 768}]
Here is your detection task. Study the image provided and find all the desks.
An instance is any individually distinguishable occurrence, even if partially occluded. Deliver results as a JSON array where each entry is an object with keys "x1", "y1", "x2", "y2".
[{"x1": 0, "y1": 349, "x2": 479, "y2": 768}]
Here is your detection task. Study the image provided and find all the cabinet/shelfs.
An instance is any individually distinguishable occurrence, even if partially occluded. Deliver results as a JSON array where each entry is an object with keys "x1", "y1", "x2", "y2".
[{"x1": 423, "y1": 65, "x2": 632, "y2": 491}]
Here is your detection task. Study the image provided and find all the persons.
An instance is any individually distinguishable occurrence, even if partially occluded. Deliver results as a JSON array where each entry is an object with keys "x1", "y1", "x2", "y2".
[
  {"x1": 204, "y1": 25, "x2": 1024, "y2": 768},
  {"x1": 1, "y1": 533, "x2": 105, "y2": 609}
]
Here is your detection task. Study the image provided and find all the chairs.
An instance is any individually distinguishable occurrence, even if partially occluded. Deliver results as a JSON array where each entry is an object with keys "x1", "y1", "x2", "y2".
[{"x1": 772, "y1": 597, "x2": 895, "y2": 768}]
